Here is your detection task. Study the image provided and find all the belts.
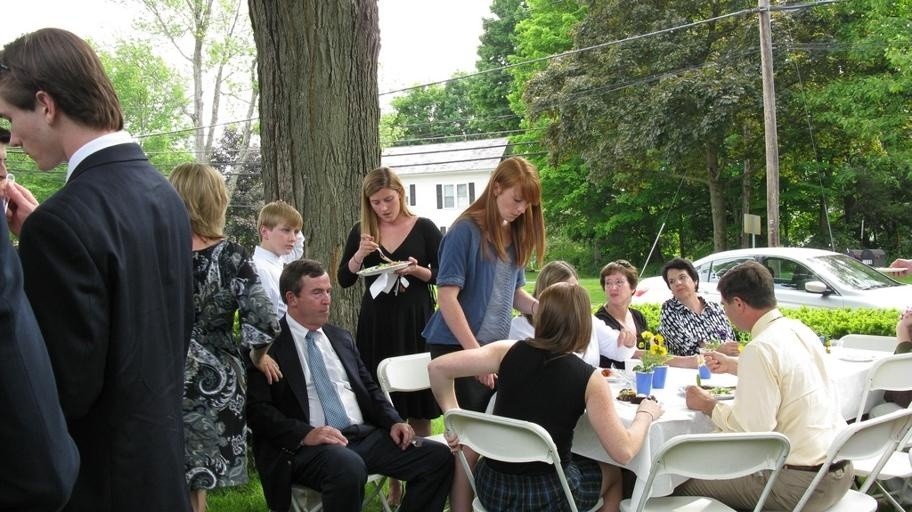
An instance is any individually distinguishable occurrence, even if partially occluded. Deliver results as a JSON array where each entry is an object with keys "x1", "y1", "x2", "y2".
[{"x1": 781, "y1": 459, "x2": 849, "y2": 472}]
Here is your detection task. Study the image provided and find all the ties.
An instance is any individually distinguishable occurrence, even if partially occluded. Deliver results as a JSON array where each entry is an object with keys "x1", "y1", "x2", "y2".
[{"x1": 305, "y1": 331, "x2": 351, "y2": 430}]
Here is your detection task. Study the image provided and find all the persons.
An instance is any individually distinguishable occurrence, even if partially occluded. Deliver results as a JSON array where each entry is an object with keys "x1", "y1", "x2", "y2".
[
  {"x1": 0, "y1": 198, "x2": 81, "y2": 512},
  {"x1": 245, "y1": 259, "x2": 456, "y2": 512},
  {"x1": 427, "y1": 281, "x2": 665, "y2": 512},
  {"x1": 508, "y1": 261, "x2": 636, "y2": 368},
  {"x1": 337, "y1": 167, "x2": 443, "y2": 512},
  {"x1": 658, "y1": 257, "x2": 740, "y2": 356},
  {"x1": 0, "y1": 28, "x2": 195, "y2": 512},
  {"x1": 851, "y1": 309, "x2": 912, "y2": 495},
  {"x1": 670, "y1": 260, "x2": 855, "y2": 512},
  {"x1": 421, "y1": 157, "x2": 545, "y2": 512},
  {"x1": 593, "y1": 260, "x2": 699, "y2": 370},
  {"x1": 167, "y1": 162, "x2": 283, "y2": 512},
  {"x1": 251, "y1": 200, "x2": 305, "y2": 322},
  {"x1": 0, "y1": 127, "x2": 11, "y2": 197}
]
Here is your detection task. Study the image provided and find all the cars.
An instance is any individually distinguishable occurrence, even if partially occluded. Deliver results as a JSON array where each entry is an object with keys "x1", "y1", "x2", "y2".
[{"x1": 631, "y1": 246, "x2": 909, "y2": 319}]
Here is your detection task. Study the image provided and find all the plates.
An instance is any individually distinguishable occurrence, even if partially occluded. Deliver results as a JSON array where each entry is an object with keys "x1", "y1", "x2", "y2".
[
  {"x1": 873, "y1": 265, "x2": 909, "y2": 278},
  {"x1": 355, "y1": 261, "x2": 414, "y2": 278},
  {"x1": 596, "y1": 366, "x2": 625, "y2": 383},
  {"x1": 617, "y1": 394, "x2": 655, "y2": 408},
  {"x1": 679, "y1": 384, "x2": 737, "y2": 401}
]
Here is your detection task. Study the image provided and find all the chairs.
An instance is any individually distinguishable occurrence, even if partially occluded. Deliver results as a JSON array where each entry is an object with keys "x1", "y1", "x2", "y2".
[
  {"x1": 845, "y1": 354, "x2": 910, "y2": 512},
  {"x1": 836, "y1": 334, "x2": 898, "y2": 366},
  {"x1": 444, "y1": 410, "x2": 604, "y2": 512},
  {"x1": 375, "y1": 352, "x2": 448, "y2": 450},
  {"x1": 289, "y1": 473, "x2": 394, "y2": 512},
  {"x1": 617, "y1": 432, "x2": 789, "y2": 512},
  {"x1": 794, "y1": 411, "x2": 911, "y2": 512}
]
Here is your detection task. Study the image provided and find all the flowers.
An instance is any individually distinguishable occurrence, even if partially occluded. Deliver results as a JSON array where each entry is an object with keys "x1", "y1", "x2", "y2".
[
  {"x1": 637, "y1": 330, "x2": 657, "y2": 370},
  {"x1": 654, "y1": 334, "x2": 672, "y2": 365}
]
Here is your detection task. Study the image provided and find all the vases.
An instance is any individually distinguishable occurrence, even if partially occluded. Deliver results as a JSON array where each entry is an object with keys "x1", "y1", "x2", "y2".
[
  {"x1": 633, "y1": 368, "x2": 654, "y2": 396},
  {"x1": 649, "y1": 365, "x2": 669, "y2": 391}
]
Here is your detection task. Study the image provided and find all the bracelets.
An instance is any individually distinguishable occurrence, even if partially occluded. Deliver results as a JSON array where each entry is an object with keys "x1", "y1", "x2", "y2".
[
  {"x1": 354, "y1": 254, "x2": 363, "y2": 265},
  {"x1": 530, "y1": 300, "x2": 537, "y2": 313},
  {"x1": 636, "y1": 411, "x2": 654, "y2": 422}
]
